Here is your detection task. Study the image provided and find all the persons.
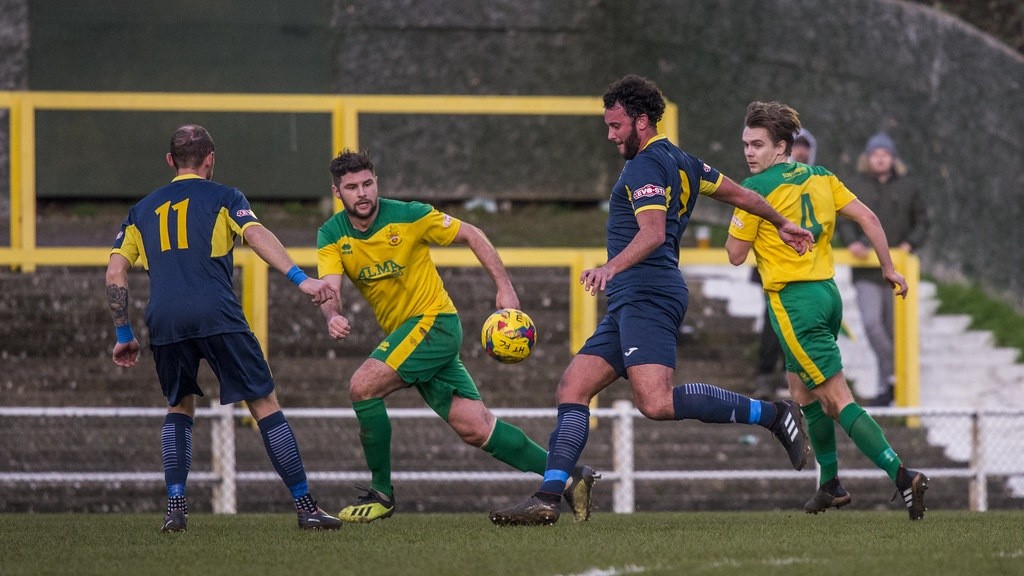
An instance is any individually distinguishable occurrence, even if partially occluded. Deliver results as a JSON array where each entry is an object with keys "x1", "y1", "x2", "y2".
[
  {"x1": 487, "y1": 72, "x2": 817, "y2": 530},
  {"x1": 754, "y1": 125, "x2": 931, "y2": 410},
  {"x1": 315, "y1": 148, "x2": 604, "y2": 524},
  {"x1": 103, "y1": 123, "x2": 347, "y2": 531},
  {"x1": 725, "y1": 98, "x2": 932, "y2": 526}
]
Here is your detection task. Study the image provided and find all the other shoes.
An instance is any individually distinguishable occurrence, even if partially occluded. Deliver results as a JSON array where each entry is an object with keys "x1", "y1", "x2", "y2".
[
  {"x1": 783, "y1": 373, "x2": 789, "y2": 388},
  {"x1": 754, "y1": 376, "x2": 775, "y2": 399},
  {"x1": 868, "y1": 384, "x2": 894, "y2": 406}
]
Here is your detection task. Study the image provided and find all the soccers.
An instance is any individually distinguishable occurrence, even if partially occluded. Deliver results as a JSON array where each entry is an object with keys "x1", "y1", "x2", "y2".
[{"x1": 481, "y1": 308, "x2": 538, "y2": 366}]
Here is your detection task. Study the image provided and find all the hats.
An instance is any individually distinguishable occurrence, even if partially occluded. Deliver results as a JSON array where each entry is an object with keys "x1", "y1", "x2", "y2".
[{"x1": 864, "y1": 131, "x2": 897, "y2": 158}]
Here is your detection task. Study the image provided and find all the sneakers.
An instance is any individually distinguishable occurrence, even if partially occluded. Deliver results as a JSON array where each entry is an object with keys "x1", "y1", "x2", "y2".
[
  {"x1": 768, "y1": 399, "x2": 810, "y2": 471},
  {"x1": 298, "y1": 500, "x2": 343, "y2": 531},
  {"x1": 562, "y1": 465, "x2": 601, "y2": 523},
  {"x1": 804, "y1": 476, "x2": 852, "y2": 515},
  {"x1": 891, "y1": 464, "x2": 930, "y2": 522},
  {"x1": 161, "y1": 509, "x2": 188, "y2": 533},
  {"x1": 489, "y1": 492, "x2": 562, "y2": 528},
  {"x1": 338, "y1": 483, "x2": 396, "y2": 523}
]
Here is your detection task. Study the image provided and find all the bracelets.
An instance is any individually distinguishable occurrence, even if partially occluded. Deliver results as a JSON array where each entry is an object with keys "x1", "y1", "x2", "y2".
[
  {"x1": 116, "y1": 324, "x2": 134, "y2": 343},
  {"x1": 285, "y1": 265, "x2": 309, "y2": 286}
]
